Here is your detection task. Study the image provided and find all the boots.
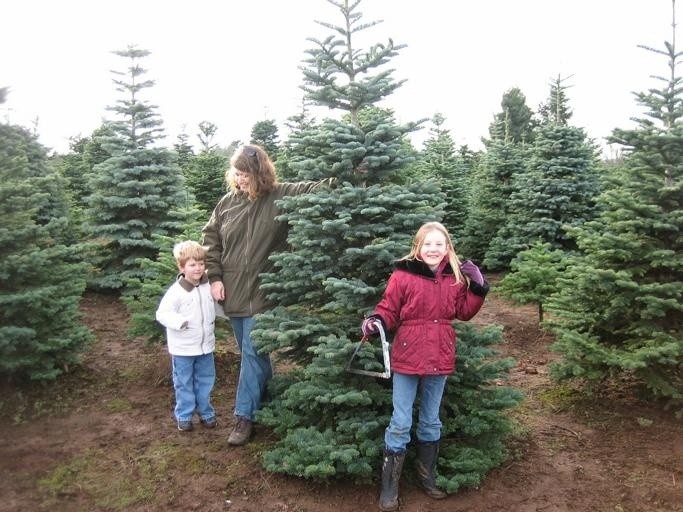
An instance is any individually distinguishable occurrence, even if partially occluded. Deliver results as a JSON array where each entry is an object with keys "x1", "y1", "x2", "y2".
[
  {"x1": 416, "y1": 440, "x2": 447, "y2": 499},
  {"x1": 378, "y1": 446, "x2": 407, "y2": 512}
]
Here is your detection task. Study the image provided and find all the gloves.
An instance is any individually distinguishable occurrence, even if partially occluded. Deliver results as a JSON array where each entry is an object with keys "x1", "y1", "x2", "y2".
[
  {"x1": 460, "y1": 261, "x2": 485, "y2": 287},
  {"x1": 361, "y1": 317, "x2": 384, "y2": 338}
]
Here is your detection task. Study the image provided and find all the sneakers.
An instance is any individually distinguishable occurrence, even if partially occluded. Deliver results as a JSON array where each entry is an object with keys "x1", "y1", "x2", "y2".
[
  {"x1": 202, "y1": 416, "x2": 217, "y2": 429},
  {"x1": 227, "y1": 418, "x2": 256, "y2": 446},
  {"x1": 177, "y1": 420, "x2": 194, "y2": 432}
]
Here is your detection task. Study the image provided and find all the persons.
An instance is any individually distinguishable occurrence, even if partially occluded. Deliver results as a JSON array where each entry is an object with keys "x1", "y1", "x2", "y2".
[
  {"x1": 360, "y1": 220, "x2": 490, "y2": 510},
  {"x1": 154, "y1": 239, "x2": 231, "y2": 431},
  {"x1": 198, "y1": 145, "x2": 337, "y2": 445}
]
